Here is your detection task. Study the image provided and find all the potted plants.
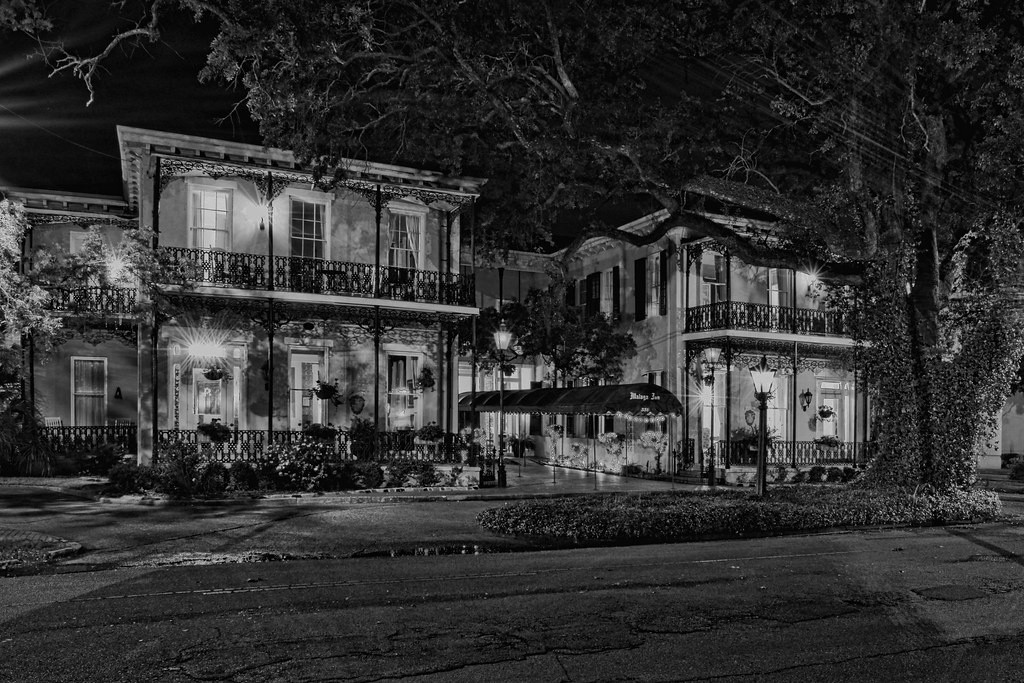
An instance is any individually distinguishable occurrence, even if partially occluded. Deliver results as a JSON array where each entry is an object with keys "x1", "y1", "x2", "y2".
[
  {"x1": 503, "y1": 431, "x2": 538, "y2": 459},
  {"x1": 298, "y1": 420, "x2": 341, "y2": 440},
  {"x1": 411, "y1": 367, "x2": 437, "y2": 393},
  {"x1": 413, "y1": 420, "x2": 445, "y2": 446},
  {"x1": 203, "y1": 365, "x2": 236, "y2": 384},
  {"x1": 815, "y1": 434, "x2": 844, "y2": 453},
  {"x1": 196, "y1": 419, "x2": 236, "y2": 444},
  {"x1": 305, "y1": 379, "x2": 345, "y2": 418},
  {"x1": 816, "y1": 405, "x2": 837, "y2": 423},
  {"x1": 742, "y1": 425, "x2": 785, "y2": 453}
]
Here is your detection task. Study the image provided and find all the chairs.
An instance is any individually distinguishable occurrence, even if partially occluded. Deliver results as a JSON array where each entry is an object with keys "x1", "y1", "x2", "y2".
[
  {"x1": 43, "y1": 416, "x2": 64, "y2": 448},
  {"x1": 115, "y1": 418, "x2": 130, "y2": 450}
]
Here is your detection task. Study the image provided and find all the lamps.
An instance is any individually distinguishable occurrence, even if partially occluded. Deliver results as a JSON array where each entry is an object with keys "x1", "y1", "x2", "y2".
[
  {"x1": 257, "y1": 198, "x2": 268, "y2": 231},
  {"x1": 802, "y1": 386, "x2": 813, "y2": 412},
  {"x1": 261, "y1": 361, "x2": 275, "y2": 390}
]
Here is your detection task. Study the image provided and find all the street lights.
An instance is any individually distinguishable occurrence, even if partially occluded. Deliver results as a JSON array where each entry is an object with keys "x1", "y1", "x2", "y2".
[
  {"x1": 491, "y1": 318, "x2": 512, "y2": 489},
  {"x1": 703, "y1": 343, "x2": 720, "y2": 486},
  {"x1": 750, "y1": 354, "x2": 777, "y2": 498}
]
